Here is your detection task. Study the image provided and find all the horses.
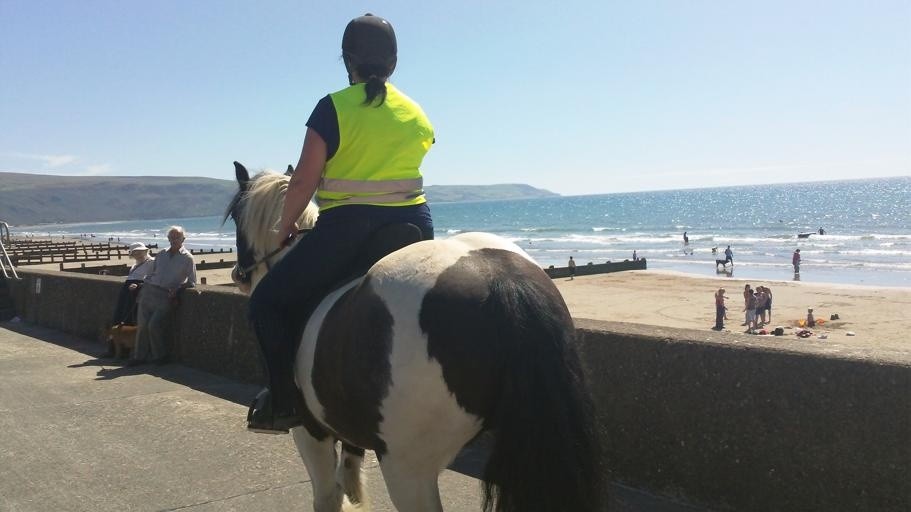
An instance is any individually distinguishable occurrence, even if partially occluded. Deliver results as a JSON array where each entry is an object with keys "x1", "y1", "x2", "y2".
[{"x1": 220, "y1": 159, "x2": 610, "y2": 512}]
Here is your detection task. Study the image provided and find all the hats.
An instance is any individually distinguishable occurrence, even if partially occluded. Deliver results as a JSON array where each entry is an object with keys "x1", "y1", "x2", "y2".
[
  {"x1": 342, "y1": 12, "x2": 398, "y2": 64},
  {"x1": 127, "y1": 242, "x2": 148, "y2": 256}
]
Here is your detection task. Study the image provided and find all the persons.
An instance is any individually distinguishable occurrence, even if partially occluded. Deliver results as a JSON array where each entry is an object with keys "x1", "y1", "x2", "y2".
[
  {"x1": 808, "y1": 307, "x2": 814, "y2": 328},
  {"x1": 793, "y1": 248, "x2": 803, "y2": 274},
  {"x1": 683, "y1": 232, "x2": 689, "y2": 244},
  {"x1": 99, "y1": 223, "x2": 198, "y2": 366},
  {"x1": 568, "y1": 255, "x2": 576, "y2": 280},
  {"x1": 743, "y1": 282, "x2": 772, "y2": 331},
  {"x1": 714, "y1": 286, "x2": 730, "y2": 329},
  {"x1": 632, "y1": 250, "x2": 637, "y2": 261},
  {"x1": 248, "y1": 11, "x2": 437, "y2": 435},
  {"x1": 818, "y1": 226, "x2": 826, "y2": 235},
  {"x1": 711, "y1": 243, "x2": 736, "y2": 270}
]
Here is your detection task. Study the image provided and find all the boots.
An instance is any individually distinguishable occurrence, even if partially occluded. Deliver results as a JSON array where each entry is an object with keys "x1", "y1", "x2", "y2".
[{"x1": 247, "y1": 300, "x2": 303, "y2": 433}]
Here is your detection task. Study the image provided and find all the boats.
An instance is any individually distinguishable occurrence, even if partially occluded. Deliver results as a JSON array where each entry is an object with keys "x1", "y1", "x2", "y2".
[{"x1": 797, "y1": 231, "x2": 816, "y2": 238}]
